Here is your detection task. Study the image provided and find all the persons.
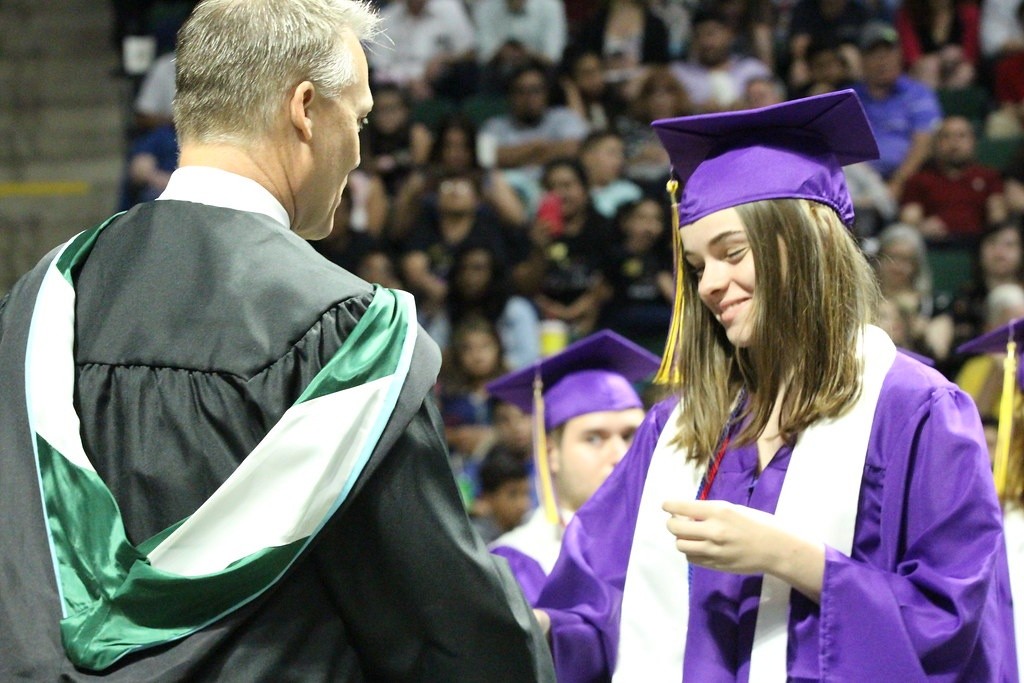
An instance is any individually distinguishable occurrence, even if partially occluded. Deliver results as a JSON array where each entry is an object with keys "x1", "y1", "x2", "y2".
[
  {"x1": 484, "y1": 331, "x2": 662, "y2": 609},
  {"x1": 118, "y1": 0, "x2": 1024, "y2": 548},
  {"x1": 0, "y1": 0, "x2": 557, "y2": 682},
  {"x1": 532, "y1": 89, "x2": 1017, "y2": 683}
]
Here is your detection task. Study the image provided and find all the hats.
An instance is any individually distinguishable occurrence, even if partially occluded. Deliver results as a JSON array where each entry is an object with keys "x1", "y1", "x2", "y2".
[
  {"x1": 488, "y1": 329, "x2": 666, "y2": 530},
  {"x1": 956, "y1": 318, "x2": 1024, "y2": 502},
  {"x1": 649, "y1": 88, "x2": 882, "y2": 387}
]
